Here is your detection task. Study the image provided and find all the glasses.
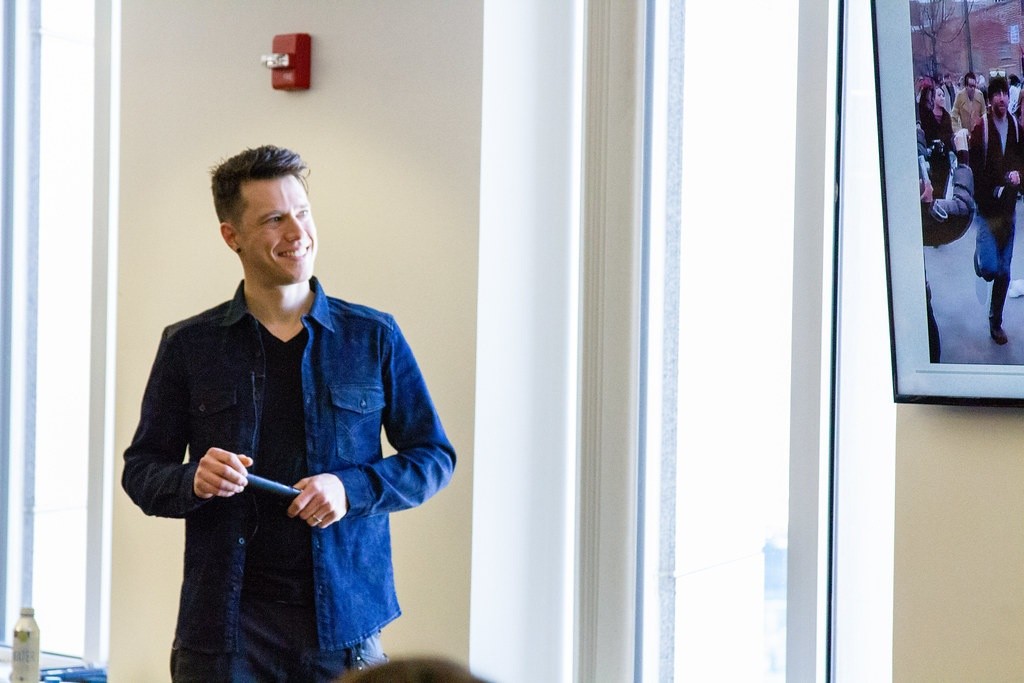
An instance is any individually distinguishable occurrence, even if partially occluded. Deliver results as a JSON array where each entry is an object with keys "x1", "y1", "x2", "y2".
[{"x1": 968, "y1": 83, "x2": 976, "y2": 89}]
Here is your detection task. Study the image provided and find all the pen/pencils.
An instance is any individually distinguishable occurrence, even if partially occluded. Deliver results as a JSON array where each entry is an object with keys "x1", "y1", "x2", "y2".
[{"x1": 246, "y1": 474, "x2": 302, "y2": 499}]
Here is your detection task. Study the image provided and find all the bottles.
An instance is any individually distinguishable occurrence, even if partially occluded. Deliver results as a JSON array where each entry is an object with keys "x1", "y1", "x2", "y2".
[{"x1": 12, "y1": 607, "x2": 40, "y2": 683}]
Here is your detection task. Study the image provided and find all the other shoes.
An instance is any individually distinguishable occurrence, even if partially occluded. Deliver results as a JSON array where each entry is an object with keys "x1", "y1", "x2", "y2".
[
  {"x1": 990, "y1": 326, "x2": 1007, "y2": 345},
  {"x1": 974, "y1": 252, "x2": 983, "y2": 277}
]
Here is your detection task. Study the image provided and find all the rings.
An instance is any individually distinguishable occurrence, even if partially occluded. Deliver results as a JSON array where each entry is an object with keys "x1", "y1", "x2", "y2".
[{"x1": 313, "y1": 513, "x2": 323, "y2": 523}]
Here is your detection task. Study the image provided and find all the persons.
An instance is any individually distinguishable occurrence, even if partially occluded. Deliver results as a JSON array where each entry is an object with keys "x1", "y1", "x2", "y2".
[
  {"x1": 120, "y1": 146, "x2": 457, "y2": 683},
  {"x1": 916, "y1": 69, "x2": 1024, "y2": 366}
]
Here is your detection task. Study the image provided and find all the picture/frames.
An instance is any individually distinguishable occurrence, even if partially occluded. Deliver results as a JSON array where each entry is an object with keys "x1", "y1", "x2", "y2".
[{"x1": 870, "y1": 0, "x2": 1024, "y2": 410}]
